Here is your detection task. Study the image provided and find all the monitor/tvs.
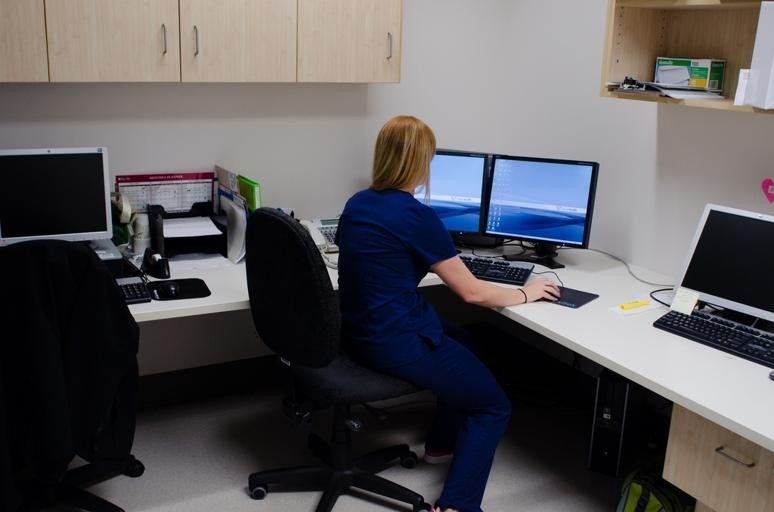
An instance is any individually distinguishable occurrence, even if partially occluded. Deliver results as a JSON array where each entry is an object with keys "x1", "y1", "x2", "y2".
[
  {"x1": 673, "y1": 204, "x2": 774, "y2": 324},
  {"x1": 415, "y1": 149, "x2": 490, "y2": 246},
  {"x1": 0, "y1": 146, "x2": 113, "y2": 248},
  {"x1": 486, "y1": 153, "x2": 600, "y2": 270}
]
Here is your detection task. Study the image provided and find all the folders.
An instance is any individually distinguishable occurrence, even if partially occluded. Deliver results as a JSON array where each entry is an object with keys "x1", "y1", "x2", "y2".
[
  {"x1": 219, "y1": 189, "x2": 247, "y2": 266},
  {"x1": 236, "y1": 174, "x2": 261, "y2": 213}
]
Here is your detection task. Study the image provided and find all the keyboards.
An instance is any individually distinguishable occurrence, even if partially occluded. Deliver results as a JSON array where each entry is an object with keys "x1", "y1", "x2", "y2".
[
  {"x1": 120, "y1": 284, "x2": 151, "y2": 305},
  {"x1": 653, "y1": 310, "x2": 769, "y2": 368},
  {"x1": 463, "y1": 255, "x2": 536, "y2": 287}
]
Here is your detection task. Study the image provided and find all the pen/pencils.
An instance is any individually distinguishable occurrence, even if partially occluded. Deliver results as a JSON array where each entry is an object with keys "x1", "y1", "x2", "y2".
[{"x1": 620, "y1": 298, "x2": 651, "y2": 311}]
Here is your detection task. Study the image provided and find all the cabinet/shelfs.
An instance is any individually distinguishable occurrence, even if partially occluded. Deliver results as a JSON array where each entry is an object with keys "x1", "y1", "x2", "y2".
[
  {"x1": 42, "y1": 1, "x2": 297, "y2": 85},
  {"x1": 599, "y1": 2, "x2": 774, "y2": 118},
  {"x1": 1, "y1": 1, "x2": 49, "y2": 83},
  {"x1": 295, "y1": 1, "x2": 402, "y2": 84}
]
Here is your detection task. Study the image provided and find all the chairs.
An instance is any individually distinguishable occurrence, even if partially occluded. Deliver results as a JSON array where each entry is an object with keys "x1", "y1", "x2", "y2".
[
  {"x1": 245, "y1": 207, "x2": 443, "y2": 512},
  {"x1": 1, "y1": 239, "x2": 145, "y2": 512}
]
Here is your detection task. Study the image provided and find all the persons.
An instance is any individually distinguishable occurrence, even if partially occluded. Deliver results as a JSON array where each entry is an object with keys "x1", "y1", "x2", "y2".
[{"x1": 333, "y1": 116, "x2": 560, "y2": 512}]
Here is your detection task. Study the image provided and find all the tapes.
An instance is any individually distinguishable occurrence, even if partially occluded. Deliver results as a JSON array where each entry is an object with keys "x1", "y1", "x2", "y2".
[{"x1": 152, "y1": 253, "x2": 163, "y2": 261}]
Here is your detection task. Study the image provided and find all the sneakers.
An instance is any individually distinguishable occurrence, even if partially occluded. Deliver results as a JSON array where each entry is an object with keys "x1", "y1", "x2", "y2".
[{"x1": 425, "y1": 446, "x2": 458, "y2": 464}]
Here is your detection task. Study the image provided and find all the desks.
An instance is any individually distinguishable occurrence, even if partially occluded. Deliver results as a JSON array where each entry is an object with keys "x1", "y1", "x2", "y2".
[
  {"x1": 122, "y1": 218, "x2": 447, "y2": 375},
  {"x1": 445, "y1": 235, "x2": 774, "y2": 512}
]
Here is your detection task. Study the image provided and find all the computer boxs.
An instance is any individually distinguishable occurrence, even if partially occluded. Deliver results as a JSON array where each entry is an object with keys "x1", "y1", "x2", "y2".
[{"x1": 587, "y1": 374, "x2": 629, "y2": 500}]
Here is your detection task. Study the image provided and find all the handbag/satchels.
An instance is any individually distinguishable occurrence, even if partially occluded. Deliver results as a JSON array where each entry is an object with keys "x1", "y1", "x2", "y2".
[{"x1": 616, "y1": 470, "x2": 695, "y2": 512}]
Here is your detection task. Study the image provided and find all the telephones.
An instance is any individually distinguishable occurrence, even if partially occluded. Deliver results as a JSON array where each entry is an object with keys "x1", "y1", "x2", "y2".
[{"x1": 296, "y1": 215, "x2": 342, "y2": 272}]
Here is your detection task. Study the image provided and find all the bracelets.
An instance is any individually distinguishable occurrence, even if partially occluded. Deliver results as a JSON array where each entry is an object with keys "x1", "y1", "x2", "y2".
[{"x1": 517, "y1": 288, "x2": 527, "y2": 303}]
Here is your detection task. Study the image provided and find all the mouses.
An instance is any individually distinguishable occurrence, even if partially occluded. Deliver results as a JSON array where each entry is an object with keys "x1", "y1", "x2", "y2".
[{"x1": 165, "y1": 281, "x2": 181, "y2": 296}]
